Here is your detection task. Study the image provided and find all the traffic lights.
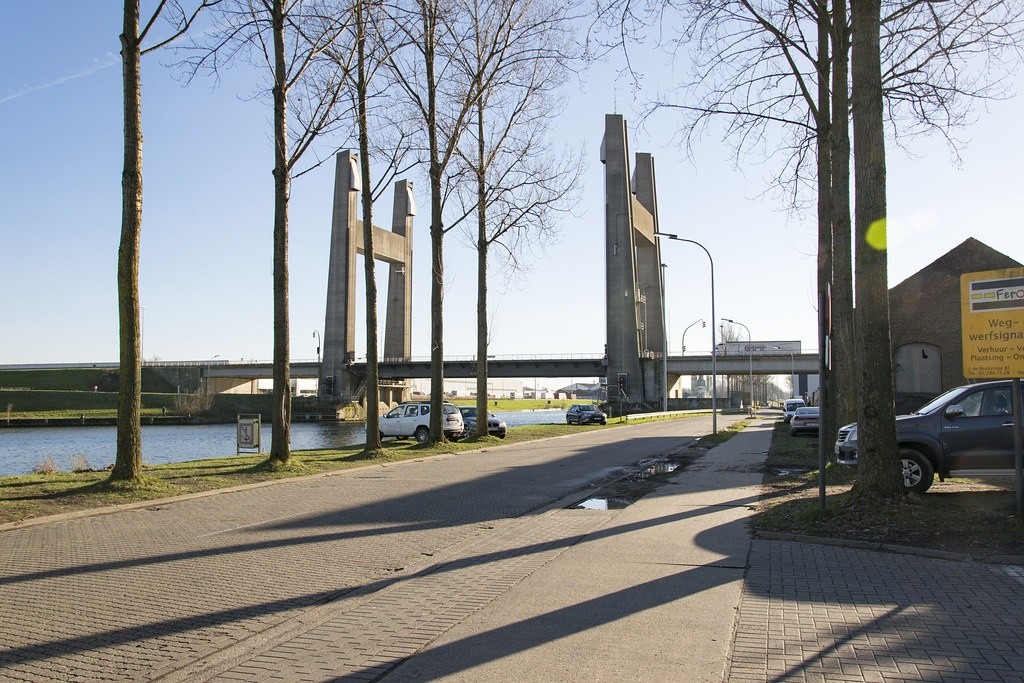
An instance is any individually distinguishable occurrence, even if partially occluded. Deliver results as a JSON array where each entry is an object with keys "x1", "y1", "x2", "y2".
[{"x1": 618, "y1": 373, "x2": 628, "y2": 394}]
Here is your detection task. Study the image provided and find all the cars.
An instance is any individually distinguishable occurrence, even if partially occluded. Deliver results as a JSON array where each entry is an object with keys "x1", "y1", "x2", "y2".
[
  {"x1": 790, "y1": 407, "x2": 819, "y2": 437},
  {"x1": 565, "y1": 403, "x2": 607, "y2": 425},
  {"x1": 457, "y1": 405, "x2": 508, "y2": 439},
  {"x1": 834, "y1": 378, "x2": 1024, "y2": 495}
]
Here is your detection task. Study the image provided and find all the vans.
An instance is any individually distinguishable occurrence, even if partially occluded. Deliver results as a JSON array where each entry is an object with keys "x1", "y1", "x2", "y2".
[{"x1": 782, "y1": 399, "x2": 805, "y2": 424}]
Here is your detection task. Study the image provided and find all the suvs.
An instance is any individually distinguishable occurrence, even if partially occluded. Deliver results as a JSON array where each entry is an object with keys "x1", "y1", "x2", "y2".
[{"x1": 364, "y1": 399, "x2": 465, "y2": 444}]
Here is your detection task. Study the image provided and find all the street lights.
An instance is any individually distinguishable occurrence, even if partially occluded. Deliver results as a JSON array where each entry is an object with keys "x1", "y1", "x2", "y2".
[
  {"x1": 721, "y1": 318, "x2": 753, "y2": 407},
  {"x1": 654, "y1": 231, "x2": 717, "y2": 436},
  {"x1": 773, "y1": 346, "x2": 794, "y2": 399}
]
